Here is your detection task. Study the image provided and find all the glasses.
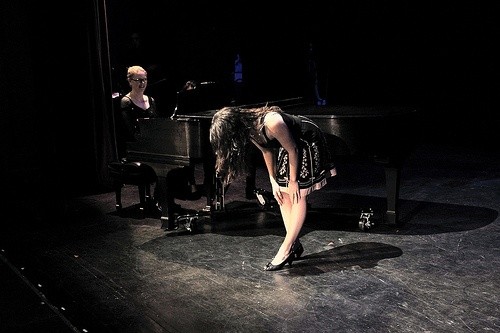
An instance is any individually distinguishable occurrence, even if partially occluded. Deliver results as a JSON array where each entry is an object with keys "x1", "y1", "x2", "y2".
[{"x1": 130, "y1": 78, "x2": 147, "y2": 82}]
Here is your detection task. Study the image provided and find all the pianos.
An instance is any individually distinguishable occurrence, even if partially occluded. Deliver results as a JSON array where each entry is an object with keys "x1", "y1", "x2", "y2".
[{"x1": 125, "y1": 80, "x2": 403, "y2": 232}]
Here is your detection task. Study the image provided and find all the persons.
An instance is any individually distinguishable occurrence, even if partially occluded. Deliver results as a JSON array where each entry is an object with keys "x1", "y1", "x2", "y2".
[
  {"x1": 120, "y1": 65, "x2": 181, "y2": 219},
  {"x1": 209, "y1": 105, "x2": 329, "y2": 271}
]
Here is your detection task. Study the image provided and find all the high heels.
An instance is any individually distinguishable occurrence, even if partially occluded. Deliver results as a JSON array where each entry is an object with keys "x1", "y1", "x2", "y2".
[
  {"x1": 263, "y1": 253, "x2": 294, "y2": 271},
  {"x1": 293, "y1": 244, "x2": 304, "y2": 258}
]
